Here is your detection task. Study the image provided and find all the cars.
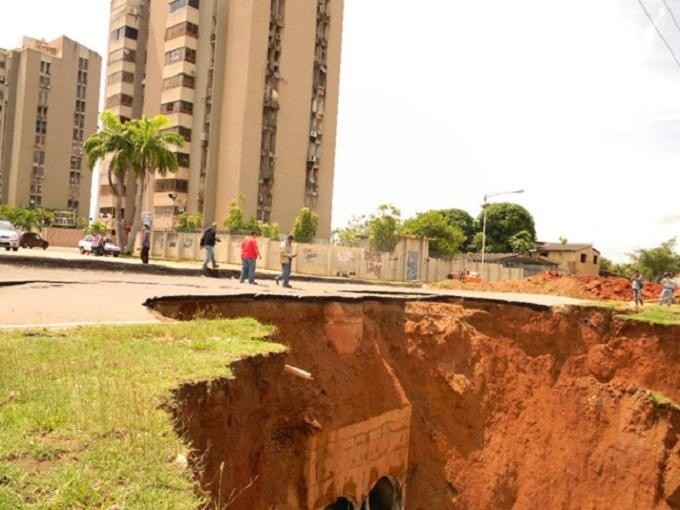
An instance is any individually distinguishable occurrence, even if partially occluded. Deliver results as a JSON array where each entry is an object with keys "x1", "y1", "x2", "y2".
[
  {"x1": 0, "y1": 220, "x2": 21, "y2": 252},
  {"x1": 78, "y1": 234, "x2": 122, "y2": 257},
  {"x1": 19, "y1": 231, "x2": 49, "y2": 250}
]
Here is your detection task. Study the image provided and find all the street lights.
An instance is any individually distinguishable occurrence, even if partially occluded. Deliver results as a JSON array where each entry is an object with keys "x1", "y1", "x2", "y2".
[{"x1": 480, "y1": 189, "x2": 525, "y2": 283}]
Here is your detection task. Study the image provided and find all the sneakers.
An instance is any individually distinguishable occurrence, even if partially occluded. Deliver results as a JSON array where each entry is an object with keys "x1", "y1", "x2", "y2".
[
  {"x1": 275, "y1": 277, "x2": 291, "y2": 288},
  {"x1": 213, "y1": 265, "x2": 218, "y2": 268}
]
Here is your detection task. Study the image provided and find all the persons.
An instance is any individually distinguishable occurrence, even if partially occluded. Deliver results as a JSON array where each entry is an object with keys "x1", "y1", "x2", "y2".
[
  {"x1": 274, "y1": 234, "x2": 299, "y2": 288},
  {"x1": 96, "y1": 231, "x2": 107, "y2": 255},
  {"x1": 239, "y1": 231, "x2": 262, "y2": 286},
  {"x1": 198, "y1": 221, "x2": 222, "y2": 271},
  {"x1": 657, "y1": 273, "x2": 677, "y2": 308},
  {"x1": 89, "y1": 232, "x2": 100, "y2": 256},
  {"x1": 139, "y1": 223, "x2": 151, "y2": 265},
  {"x1": 630, "y1": 270, "x2": 644, "y2": 307}
]
